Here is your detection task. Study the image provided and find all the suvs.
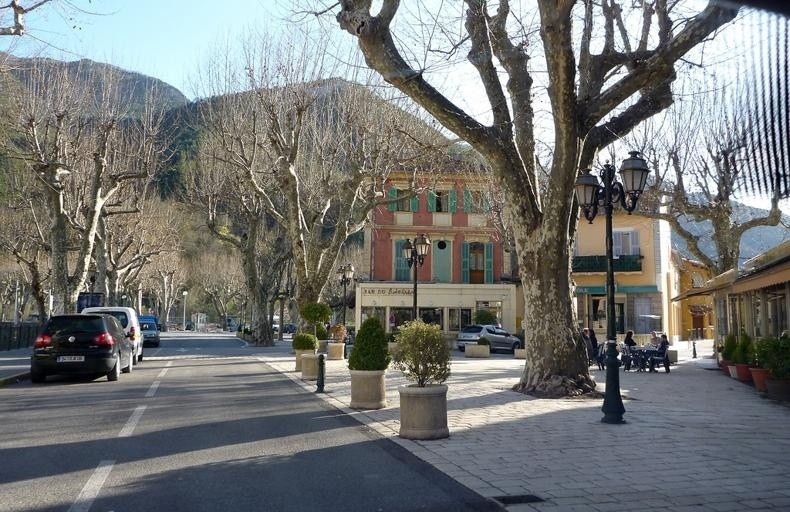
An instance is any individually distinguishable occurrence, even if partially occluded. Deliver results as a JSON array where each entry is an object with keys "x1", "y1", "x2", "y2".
[{"x1": 456, "y1": 323, "x2": 522, "y2": 355}]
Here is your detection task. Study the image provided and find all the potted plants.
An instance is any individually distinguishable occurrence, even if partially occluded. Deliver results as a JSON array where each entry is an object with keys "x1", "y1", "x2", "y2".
[
  {"x1": 721, "y1": 332, "x2": 789, "y2": 404},
  {"x1": 235, "y1": 325, "x2": 256, "y2": 343},
  {"x1": 345, "y1": 315, "x2": 393, "y2": 411},
  {"x1": 384, "y1": 318, "x2": 453, "y2": 441},
  {"x1": 292, "y1": 299, "x2": 346, "y2": 381}
]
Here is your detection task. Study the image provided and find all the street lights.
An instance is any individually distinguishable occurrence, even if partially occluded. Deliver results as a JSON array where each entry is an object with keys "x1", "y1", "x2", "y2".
[
  {"x1": 120, "y1": 293, "x2": 127, "y2": 306},
  {"x1": 182, "y1": 289, "x2": 189, "y2": 331},
  {"x1": 572, "y1": 151, "x2": 652, "y2": 425},
  {"x1": 402, "y1": 230, "x2": 431, "y2": 321},
  {"x1": 336, "y1": 263, "x2": 356, "y2": 360}
]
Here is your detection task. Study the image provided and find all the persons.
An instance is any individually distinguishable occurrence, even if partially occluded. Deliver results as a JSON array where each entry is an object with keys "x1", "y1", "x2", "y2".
[
  {"x1": 625, "y1": 331, "x2": 640, "y2": 368},
  {"x1": 641, "y1": 335, "x2": 668, "y2": 372},
  {"x1": 582, "y1": 328, "x2": 598, "y2": 360},
  {"x1": 649, "y1": 330, "x2": 661, "y2": 348}
]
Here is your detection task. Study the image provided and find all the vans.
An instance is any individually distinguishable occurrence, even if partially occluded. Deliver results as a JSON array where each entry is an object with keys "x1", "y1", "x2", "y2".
[{"x1": 82, "y1": 307, "x2": 145, "y2": 364}]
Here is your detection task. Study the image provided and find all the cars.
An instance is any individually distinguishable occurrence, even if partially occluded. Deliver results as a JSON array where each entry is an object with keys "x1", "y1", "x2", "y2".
[
  {"x1": 138, "y1": 318, "x2": 161, "y2": 348},
  {"x1": 217, "y1": 309, "x2": 299, "y2": 339},
  {"x1": 26, "y1": 313, "x2": 134, "y2": 383}
]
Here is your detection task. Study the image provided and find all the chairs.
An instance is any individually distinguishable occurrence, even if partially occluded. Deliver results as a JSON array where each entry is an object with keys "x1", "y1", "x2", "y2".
[{"x1": 595, "y1": 340, "x2": 672, "y2": 374}]
